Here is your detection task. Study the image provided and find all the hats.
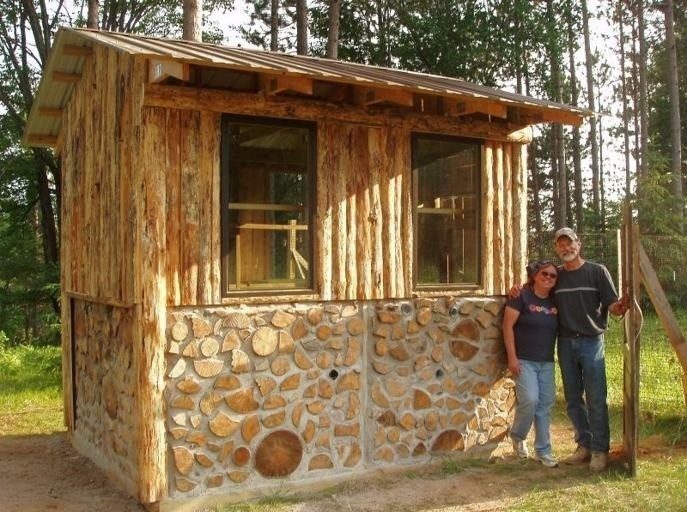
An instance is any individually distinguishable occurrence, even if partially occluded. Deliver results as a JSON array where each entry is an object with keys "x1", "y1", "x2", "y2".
[{"x1": 553, "y1": 228, "x2": 577, "y2": 243}]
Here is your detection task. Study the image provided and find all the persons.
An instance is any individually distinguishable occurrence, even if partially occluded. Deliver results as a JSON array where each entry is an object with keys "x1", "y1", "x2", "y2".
[
  {"x1": 502, "y1": 261, "x2": 560, "y2": 467},
  {"x1": 507, "y1": 228, "x2": 627, "y2": 471}
]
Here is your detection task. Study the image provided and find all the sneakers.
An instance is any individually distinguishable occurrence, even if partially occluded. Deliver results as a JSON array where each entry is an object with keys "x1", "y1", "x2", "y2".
[
  {"x1": 536, "y1": 454, "x2": 559, "y2": 468},
  {"x1": 589, "y1": 450, "x2": 608, "y2": 473},
  {"x1": 513, "y1": 439, "x2": 528, "y2": 459},
  {"x1": 565, "y1": 445, "x2": 591, "y2": 465}
]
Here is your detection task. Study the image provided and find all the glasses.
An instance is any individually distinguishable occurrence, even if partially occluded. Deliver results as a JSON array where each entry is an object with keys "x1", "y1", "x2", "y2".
[{"x1": 540, "y1": 269, "x2": 558, "y2": 279}]
